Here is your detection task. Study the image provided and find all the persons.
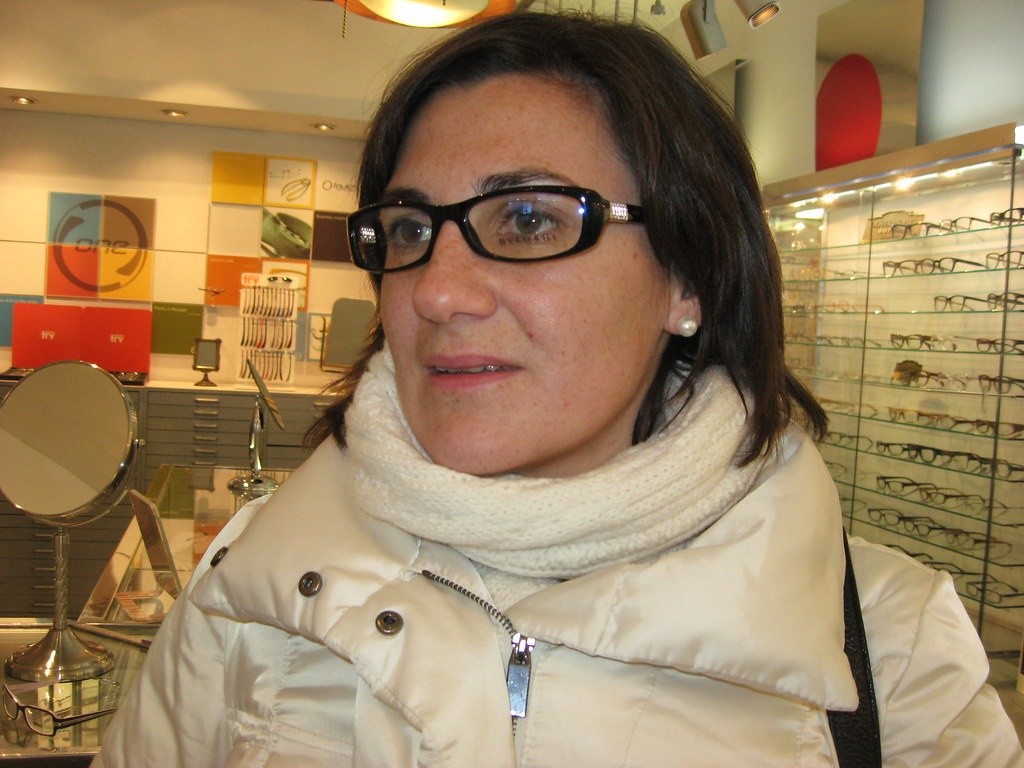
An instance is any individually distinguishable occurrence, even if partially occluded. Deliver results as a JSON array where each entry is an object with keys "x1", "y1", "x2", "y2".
[{"x1": 86, "y1": 8, "x2": 1024, "y2": 767}]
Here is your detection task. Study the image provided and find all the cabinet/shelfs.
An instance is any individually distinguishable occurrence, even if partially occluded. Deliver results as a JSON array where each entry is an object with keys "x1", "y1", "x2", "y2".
[
  {"x1": 785, "y1": 210, "x2": 1021, "y2": 623},
  {"x1": 0, "y1": 367, "x2": 350, "y2": 620}
]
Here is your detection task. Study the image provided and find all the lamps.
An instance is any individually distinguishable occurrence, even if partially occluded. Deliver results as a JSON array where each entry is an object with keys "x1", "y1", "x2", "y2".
[
  {"x1": 332, "y1": 0, "x2": 519, "y2": 39},
  {"x1": 733, "y1": 0, "x2": 779, "y2": 30},
  {"x1": 681, "y1": 0, "x2": 727, "y2": 61}
]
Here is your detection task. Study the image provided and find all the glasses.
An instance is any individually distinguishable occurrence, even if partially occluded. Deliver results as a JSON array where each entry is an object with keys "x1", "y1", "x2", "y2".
[{"x1": 346, "y1": 184, "x2": 652, "y2": 274}]
